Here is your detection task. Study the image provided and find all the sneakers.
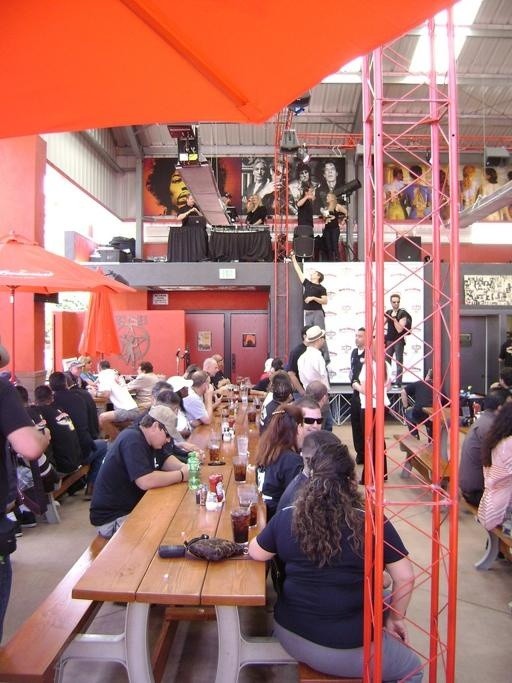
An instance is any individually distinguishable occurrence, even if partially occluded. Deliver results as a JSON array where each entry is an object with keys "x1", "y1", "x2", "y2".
[
  {"x1": 20, "y1": 511, "x2": 37, "y2": 527},
  {"x1": 12, "y1": 521, "x2": 23, "y2": 537}
]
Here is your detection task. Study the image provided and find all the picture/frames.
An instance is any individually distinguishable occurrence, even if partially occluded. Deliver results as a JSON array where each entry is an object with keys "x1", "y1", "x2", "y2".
[
  {"x1": 243, "y1": 333, "x2": 256, "y2": 347},
  {"x1": 198, "y1": 330, "x2": 212, "y2": 352}
]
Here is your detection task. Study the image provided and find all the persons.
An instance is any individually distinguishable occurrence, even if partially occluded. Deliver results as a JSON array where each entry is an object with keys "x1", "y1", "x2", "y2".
[
  {"x1": 246, "y1": 195, "x2": 266, "y2": 225},
  {"x1": 296, "y1": 187, "x2": 313, "y2": 262},
  {"x1": 1, "y1": 375, "x2": 50, "y2": 643},
  {"x1": 498, "y1": 331, "x2": 511, "y2": 367},
  {"x1": 177, "y1": 194, "x2": 204, "y2": 227},
  {"x1": 15, "y1": 357, "x2": 159, "y2": 536},
  {"x1": 296, "y1": 326, "x2": 334, "y2": 429},
  {"x1": 385, "y1": 294, "x2": 412, "y2": 386},
  {"x1": 353, "y1": 338, "x2": 391, "y2": 485},
  {"x1": 459, "y1": 367, "x2": 512, "y2": 561},
  {"x1": 89, "y1": 405, "x2": 191, "y2": 540},
  {"x1": 222, "y1": 193, "x2": 240, "y2": 227},
  {"x1": 323, "y1": 193, "x2": 347, "y2": 261},
  {"x1": 148, "y1": 157, "x2": 191, "y2": 216},
  {"x1": 253, "y1": 358, "x2": 341, "y2": 511},
  {"x1": 286, "y1": 326, "x2": 315, "y2": 396},
  {"x1": 248, "y1": 442, "x2": 422, "y2": 683},
  {"x1": 289, "y1": 250, "x2": 331, "y2": 369},
  {"x1": 138, "y1": 355, "x2": 230, "y2": 467},
  {"x1": 242, "y1": 160, "x2": 338, "y2": 215},
  {"x1": 350, "y1": 328, "x2": 380, "y2": 464},
  {"x1": 385, "y1": 164, "x2": 512, "y2": 222}
]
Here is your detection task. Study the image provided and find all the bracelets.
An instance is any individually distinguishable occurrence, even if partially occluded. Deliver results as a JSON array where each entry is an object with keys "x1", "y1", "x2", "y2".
[{"x1": 180, "y1": 470, "x2": 184, "y2": 482}]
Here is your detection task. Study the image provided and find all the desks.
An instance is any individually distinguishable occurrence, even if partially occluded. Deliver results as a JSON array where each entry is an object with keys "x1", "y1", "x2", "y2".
[
  {"x1": 208, "y1": 230, "x2": 274, "y2": 262},
  {"x1": 166, "y1": 225, "x2": 208, "y2": 262},
  {"x1": 109, "y1": 238, "x2": 135, "y2": 262},
  {"x1": 327, "y1": 385, "x2": 416, "y2": 426}
]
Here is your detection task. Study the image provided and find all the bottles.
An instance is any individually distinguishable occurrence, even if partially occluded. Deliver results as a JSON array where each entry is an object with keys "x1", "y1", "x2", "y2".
[{"x1": 188, "y1": 453, "x2": 207, "y2": 506}]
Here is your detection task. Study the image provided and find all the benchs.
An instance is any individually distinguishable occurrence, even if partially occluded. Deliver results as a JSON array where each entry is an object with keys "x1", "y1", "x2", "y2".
[
  {"x1": 458, "y1": 492, "x2": 512, "y2": 571},
  {"x1": 392, "y1": 433, "x2": 449, "y2": 513},
  {"x1": 0, "y1": 531, "x2": 113, "y2": 683},
  {"x1": 43, "y1": 460, "x2": 92, "y2": 524},
  {"x1": 297, "y1": 660, "x2": 366, "y2": 683}
]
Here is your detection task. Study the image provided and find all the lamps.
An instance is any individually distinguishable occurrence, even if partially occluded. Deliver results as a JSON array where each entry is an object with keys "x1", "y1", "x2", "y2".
[
  {"x1": 353, "y1": 138, "x2": 374, "y2": 166},
  {"x1": 287, "y1": 91, "x2": 311, "y2": 107},
  {"x1": 485, "y1": 145, "x2": 510, "y2": 167},
  {"x1": 281, "y1": 129, "x2": 299, "y2": 151}
]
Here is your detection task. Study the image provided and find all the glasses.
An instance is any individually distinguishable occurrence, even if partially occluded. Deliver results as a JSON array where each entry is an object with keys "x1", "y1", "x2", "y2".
[{"x1": 305, "y1": 418, "x2": 324, "y2": 424}]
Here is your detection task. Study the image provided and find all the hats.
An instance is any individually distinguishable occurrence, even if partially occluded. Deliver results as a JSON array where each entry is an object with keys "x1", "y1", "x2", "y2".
[
  {"x1": 148, "y1": 405, "x2": 182, "y2": 439},
  {"x1": 71, "y1": 361, "x2": 85, "y2": 368},
  {"x1": 305, "y1": 326, "x2": 325, "y2": 343},
  {"x1": 166, "y1": 376, "x2": 193, "y2": 392}
]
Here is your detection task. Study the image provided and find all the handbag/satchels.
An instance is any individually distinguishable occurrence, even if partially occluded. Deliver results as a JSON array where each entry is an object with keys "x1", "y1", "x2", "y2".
[{"x1": 187, "y1": 534, "x2": 245, "y2": 561}]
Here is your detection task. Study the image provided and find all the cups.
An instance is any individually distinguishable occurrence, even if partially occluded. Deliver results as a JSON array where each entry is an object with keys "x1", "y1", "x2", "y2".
[{"x1": 208, "y1": 376, "x2": 260, "y2": 546}]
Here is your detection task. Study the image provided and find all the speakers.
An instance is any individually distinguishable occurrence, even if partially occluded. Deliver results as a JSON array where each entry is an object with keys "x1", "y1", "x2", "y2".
[{"x1": 395, "y1": 237, "x2": 421, "y2": 264}]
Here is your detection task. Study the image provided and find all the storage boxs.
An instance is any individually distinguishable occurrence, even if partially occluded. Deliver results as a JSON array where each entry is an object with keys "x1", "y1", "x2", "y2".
[
  {"x1": 187, "y1": 215, "x2": 206, "y2": 228},
  {"x1": 96, "y1": 250, "x2": 128, "y2": 263},
  {"x1": 89, "y1": 255, "x2": 101, "y2": 262}
]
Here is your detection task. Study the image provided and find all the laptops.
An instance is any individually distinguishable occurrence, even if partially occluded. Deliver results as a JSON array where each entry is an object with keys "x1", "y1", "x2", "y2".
[{"x1": 188, "y1": 216, "x2": 206, "y2": 227}]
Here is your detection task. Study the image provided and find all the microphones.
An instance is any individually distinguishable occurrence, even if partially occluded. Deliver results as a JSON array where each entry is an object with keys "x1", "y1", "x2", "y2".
[
  {"x1": 183, "y1": 350, "x2": 188, "y2": 358},
  {"x1": 176, "y1": 348, "x2": 181, "y2": 356}
]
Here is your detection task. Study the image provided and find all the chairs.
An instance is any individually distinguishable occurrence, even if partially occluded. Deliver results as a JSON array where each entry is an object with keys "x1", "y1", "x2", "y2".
[{"x1": 293, "y1": 224, "x2": 314, "y2": 261}]
